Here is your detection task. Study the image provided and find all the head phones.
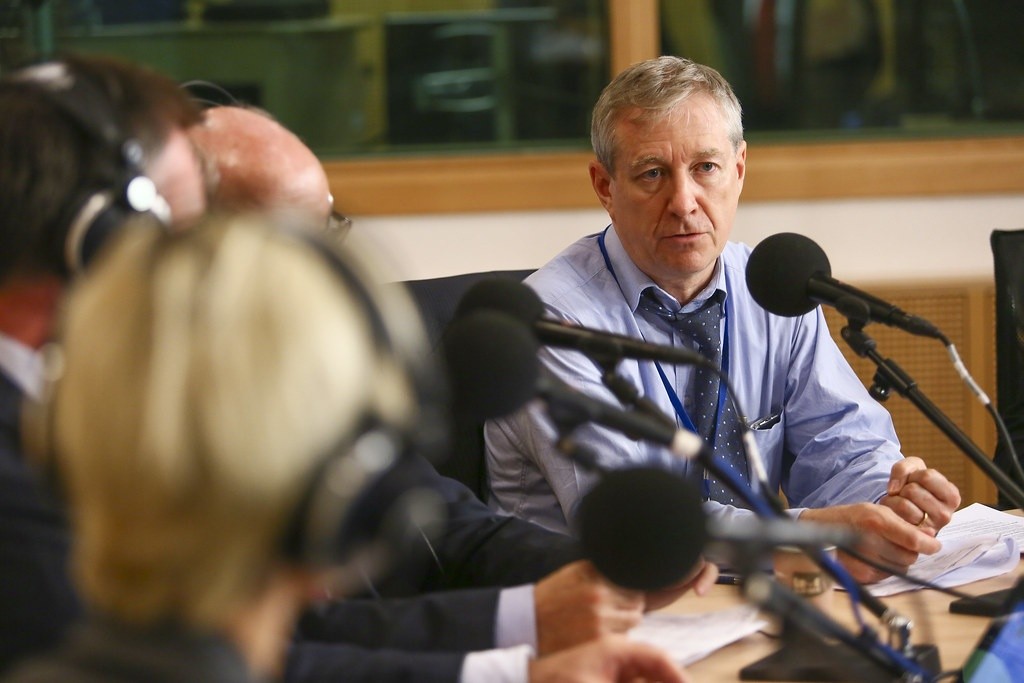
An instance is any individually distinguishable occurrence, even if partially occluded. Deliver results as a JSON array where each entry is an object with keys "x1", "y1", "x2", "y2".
[
  {"x1": 13, "y1": 58, "x2": 173, "y2": 281},
  {"x1": 23, "y1": 210, "x2": 444, "y2": 580}
]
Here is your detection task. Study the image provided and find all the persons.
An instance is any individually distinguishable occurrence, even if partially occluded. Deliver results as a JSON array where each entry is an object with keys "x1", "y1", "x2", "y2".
[
  {"x1": 0, "y1": 57, "x2": 718, "y2": 683},
  {"x1": 482, "y1": 55, "x2": 962, "y2": 585}
]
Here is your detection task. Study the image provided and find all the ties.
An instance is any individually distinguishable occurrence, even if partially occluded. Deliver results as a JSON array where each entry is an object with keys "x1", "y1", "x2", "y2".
[{"x1": 634, "y1": 291, "x2": 754, "y2": 571}]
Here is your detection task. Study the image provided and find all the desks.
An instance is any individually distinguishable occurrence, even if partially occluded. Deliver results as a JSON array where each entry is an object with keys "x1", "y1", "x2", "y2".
[{"x1": 613, "y1": 506, "x2": 1024, "y2": 682}]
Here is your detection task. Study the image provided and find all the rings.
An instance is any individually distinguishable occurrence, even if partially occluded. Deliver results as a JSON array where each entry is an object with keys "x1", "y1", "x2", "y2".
[{"x1": 915, "y1": 512, "x2": 928, "y2": 527}]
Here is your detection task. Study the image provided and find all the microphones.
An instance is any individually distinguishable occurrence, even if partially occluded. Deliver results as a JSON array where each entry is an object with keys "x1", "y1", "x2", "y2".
[
  {"x1": 447, "y1": 280, "x2": 864, "y2": 590},
  {"x1": 745, "y1": 232, "x2": 939, "y2": 336}
]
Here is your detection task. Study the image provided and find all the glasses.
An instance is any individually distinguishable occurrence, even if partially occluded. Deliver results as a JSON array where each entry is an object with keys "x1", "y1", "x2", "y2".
[{"x1": 324, "y1": 212, "x2": 352, "y2": 249}]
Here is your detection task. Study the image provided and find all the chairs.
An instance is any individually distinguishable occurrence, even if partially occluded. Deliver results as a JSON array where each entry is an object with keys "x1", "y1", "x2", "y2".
[{"x1": 390, "y1": 269, "x2": 537, "y2": 492}]
